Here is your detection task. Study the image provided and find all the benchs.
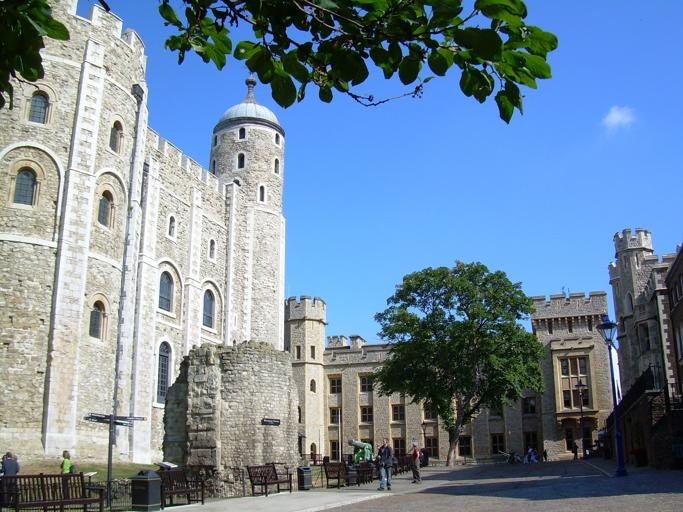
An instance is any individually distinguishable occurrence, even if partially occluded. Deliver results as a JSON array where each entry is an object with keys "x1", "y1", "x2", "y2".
[
  {"x1": 248, "y1": 464, "x2": 292, "y2": 497},
  {"x1": 0, "y1": 472, "x2": 106, "y2": 512},
  {"x1": 156, "y1": 471, "x2": 204, "y2": 509},
  {"x1": 325, "y1": 454, "x2": 411, "y2": 488}
]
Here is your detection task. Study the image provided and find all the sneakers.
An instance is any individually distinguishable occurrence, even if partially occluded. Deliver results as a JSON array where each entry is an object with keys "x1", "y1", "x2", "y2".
[
  {"x1": 377, "y1": 486, "x2": 392, "y2": 491},
  {"x1": 410, "y1": 479, "x2": 423, "y2": 484}
]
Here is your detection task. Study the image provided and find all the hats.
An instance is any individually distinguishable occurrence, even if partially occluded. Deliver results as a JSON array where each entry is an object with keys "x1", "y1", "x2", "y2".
[{"x1": 412, "y1": 442, "x2": 418, "y2": 447}]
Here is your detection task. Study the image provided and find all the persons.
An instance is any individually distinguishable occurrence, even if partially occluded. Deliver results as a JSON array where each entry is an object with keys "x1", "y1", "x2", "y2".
[
  {"x1": 378, "y1": 438, "x2": 393, "y2": 491},
  {"x1": 507, "y1": 450, "x2": 515, "y2": 464},
  {"x1": 593, "y1": 440, "x2": 600, "y2": 456},
  {"x1": 543, "y1": 449, "x2": 547, "y2": 461},
  {"x1": 570, "y1": 443, "x2": 578, "y2": 460},
  {"x1": 61, "y1": 450, "x2": 74, "y2": 500},
  {"x1": 402, "y1": 454, "x2": 408, "y2": 467},
  {"x1": 411, "y1": 443, "x2": 422, "y2": 484},
  {"x1": 0, "y1": 451, "x2": 21, "y2": 507},
  {"x1": 524, "y1": 446, "x2": 539, "y2": 465}
]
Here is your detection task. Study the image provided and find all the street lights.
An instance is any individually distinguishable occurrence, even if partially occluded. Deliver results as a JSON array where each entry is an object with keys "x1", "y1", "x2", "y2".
[
  {"x1": 419, "y1": 420, "x2": 428, "y2": 448},
  {"x1": 593, "y1": 309, "x2": 630, "y2": 478},
  {"x1": 573, "y1": 378, "x2": 587, "y2": 457}
]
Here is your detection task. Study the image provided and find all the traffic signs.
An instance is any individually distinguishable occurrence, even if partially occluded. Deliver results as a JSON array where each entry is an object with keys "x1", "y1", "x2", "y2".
[{"x1": 82, "y1": 412, "x2": 147, "y2": 428}]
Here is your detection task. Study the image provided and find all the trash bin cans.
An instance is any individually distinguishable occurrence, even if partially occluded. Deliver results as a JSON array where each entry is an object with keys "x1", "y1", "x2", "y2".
[
  {"x1": 297, "y1": 467, "x2": 312, "y2": 491},
  {"x1": 131, "y1": 469, "x2": 162, "y2": 512}
]
